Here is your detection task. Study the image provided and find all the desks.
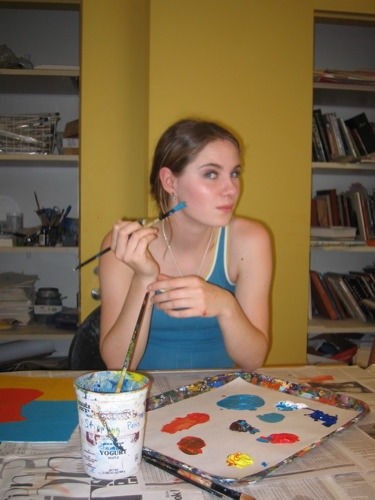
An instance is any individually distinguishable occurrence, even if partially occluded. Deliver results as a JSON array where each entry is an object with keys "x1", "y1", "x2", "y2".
[{"x1": 0, "y1": 365, "x2": 375, "y2": 500}]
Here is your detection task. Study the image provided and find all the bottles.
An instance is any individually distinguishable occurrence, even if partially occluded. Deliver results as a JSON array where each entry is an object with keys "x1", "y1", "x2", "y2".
[{"x1": 39, "y1": 226, "x2": 50, "y2": 246}]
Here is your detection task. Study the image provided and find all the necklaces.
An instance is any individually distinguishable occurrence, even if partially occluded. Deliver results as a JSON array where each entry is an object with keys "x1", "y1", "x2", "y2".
[{"x1": 159, "y1": 217, "x2": 217, "y2": 281}]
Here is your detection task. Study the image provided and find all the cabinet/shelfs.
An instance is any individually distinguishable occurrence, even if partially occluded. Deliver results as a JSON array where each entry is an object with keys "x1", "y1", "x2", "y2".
[
  {"x1": 0, "y1": 0, "x2": 148, "y2": 372},
  {"x1": 148, "y1": 0, "x2": 374, "y2": 364}
]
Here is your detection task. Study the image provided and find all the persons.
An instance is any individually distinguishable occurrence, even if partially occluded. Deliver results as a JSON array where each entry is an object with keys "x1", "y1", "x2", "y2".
[{"x1": 98, "y1": 117, "x2": 273, "y2": 370}]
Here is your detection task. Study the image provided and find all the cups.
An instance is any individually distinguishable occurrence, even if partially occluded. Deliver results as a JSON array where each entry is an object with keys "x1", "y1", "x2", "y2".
[
  {"x1": 7, "y1": 214, "x2": 22, "y2": 230},
  {"x1": 73, "y1": 371, "x2": 150, "y2": 479}
]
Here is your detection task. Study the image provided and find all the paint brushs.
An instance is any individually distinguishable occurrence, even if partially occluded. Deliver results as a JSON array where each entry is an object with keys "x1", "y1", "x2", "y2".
[
  {"x1": 0, "y1": 111, "x2": 61, "y2": 153},
  {"x1": 73, "y1": 202, "x2": 185, "y2": 271},
  {"x1": 33, "y1": 191, "x2": 72, "y2": 228},
  {"x1": 140, "y1": 447, "x2": 255, "y2": 500},
  {"x1": 114, "y1": 291, "x2": 151, "y2": 392}
]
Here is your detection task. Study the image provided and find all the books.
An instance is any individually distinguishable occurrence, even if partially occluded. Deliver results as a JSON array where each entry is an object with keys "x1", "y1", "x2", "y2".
[
  {"x1": 311, "y1": 69, "x2": 375, "y2": 326},
  {"x1": 0, "y1": 273, "x2": 36, "y2": 328}
]
